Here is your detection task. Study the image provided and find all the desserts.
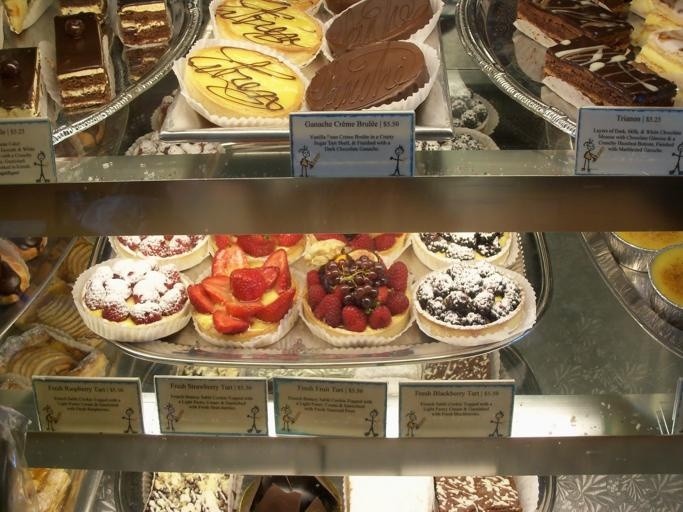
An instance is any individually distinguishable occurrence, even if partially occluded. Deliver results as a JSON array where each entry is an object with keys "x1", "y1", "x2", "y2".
[{"x1": 82, "y1": 234, "x2": 526, "y2": 349}]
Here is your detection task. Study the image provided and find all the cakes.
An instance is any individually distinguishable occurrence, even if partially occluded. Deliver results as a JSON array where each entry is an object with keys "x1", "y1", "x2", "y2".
[
  {"x1": 512, "y1": 0, "x2": 683, "y2": 107},
  {"x1": 183, "y1": 1, "x2": 434, "y2": 115},
  {"x1": 0, "y1": 237, "x2": 96, "y2": 509},
  {"x1": 606, "y1": 230, "x2": 683, "y2": 328},
  {"x1": 117, "y1": 471, "x2": 524, "y2": 512},
  {"x1": 0, "y1": 0, "x2": 175, "y2": 132}
]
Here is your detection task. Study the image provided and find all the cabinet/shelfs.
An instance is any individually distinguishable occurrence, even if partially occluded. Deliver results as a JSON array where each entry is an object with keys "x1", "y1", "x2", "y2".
[{"x1": 0, "y1": 0, "x2": 683, "y2": 510}]
